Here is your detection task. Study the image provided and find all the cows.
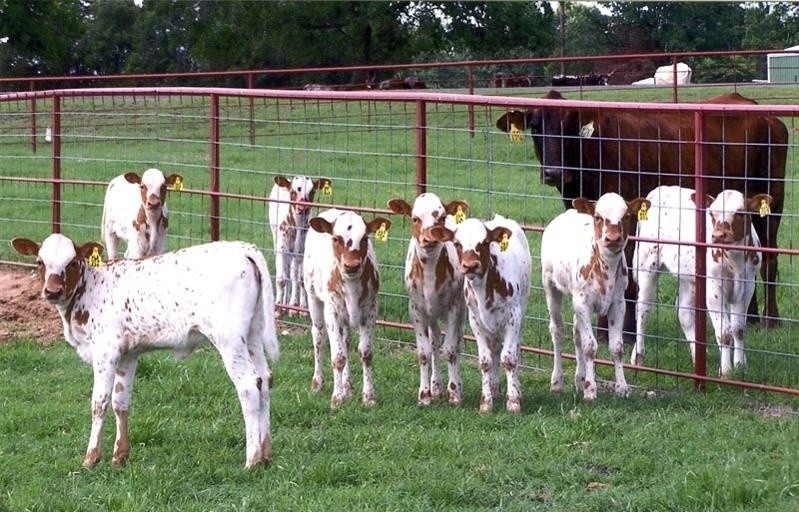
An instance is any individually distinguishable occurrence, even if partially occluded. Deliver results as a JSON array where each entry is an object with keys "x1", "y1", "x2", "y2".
[
  {"x1": 538, "y1": 189, "x2": 653, "y2": 403},
  {"x1": 652, "y1": 61, "x2": 693, "y2": 88},
  {"x1": 300, "y1": 205, "x2": 392, "y2": 413},
  {"x1": 427, "y1": 214, "x2": 534, "y2": 415},
  {"x1": 267, "y1": 173, "x2": 332, "y2": 321},
  {"x1": 386, "y1": 192, "x2": 473, "y2": 411},
  {"x1": 495, "y1": 87, "x2": 792, "y2": 343},
  {"x1": 11, "y1": 232, "x2": 285, "y2": 469},
  {"x1": 495, "y1": 71, "x2": 533, "y2": 87},
  {"x1": 628, "y1": 182, "x2": 776, "y2": 376},
  {"x1": 552, "y1": 74, "x2": 609, "y2": 87},
  {"x1": 100, "y1": 169, "x2": 184, "y2": 262}
]
